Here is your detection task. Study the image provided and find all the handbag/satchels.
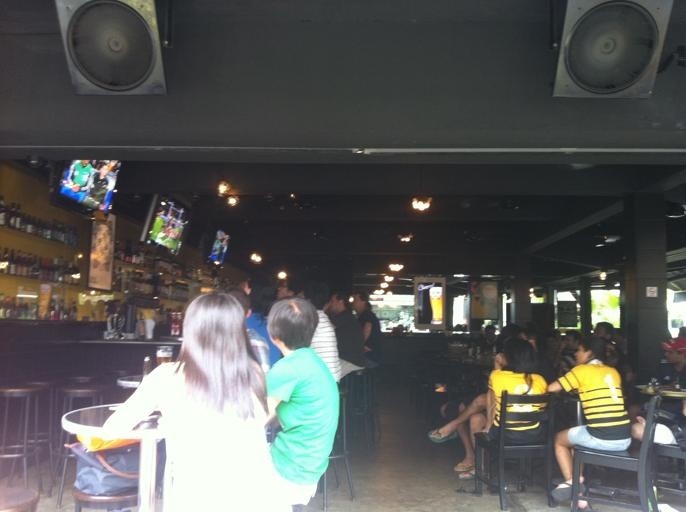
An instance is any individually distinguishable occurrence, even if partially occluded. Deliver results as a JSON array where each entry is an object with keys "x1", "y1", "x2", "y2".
[{"x1": 63, "y1": 434, "x2": 165, "y2": 497}]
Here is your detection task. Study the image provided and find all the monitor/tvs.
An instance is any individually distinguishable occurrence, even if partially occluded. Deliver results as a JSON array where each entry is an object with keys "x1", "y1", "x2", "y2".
[
  {"x1": 206, "y1": 228, "x2": 231, "y2": 269},
  {"x1": 139, "y1": 191, "x2": 193, "y2": 257},
  {"x1": 49, "y1": 159, "x2": 122, "y2": 221}
]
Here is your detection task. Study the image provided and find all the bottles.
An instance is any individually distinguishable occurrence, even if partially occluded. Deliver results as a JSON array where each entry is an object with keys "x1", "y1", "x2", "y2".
[
  {"x1": 0, "y1": 247, "x2": 78, "y2": 285},
  {"x1": 111, "y1": 241, "x2": 202, "y2": 300},
  {"x1": 0, "y1": 196, "x2": 80, "y2": 244},
  {"x1": 142, "y1": 353, "x2": 151, "y2": 378},
  {"x1": 0, "y1": 292, "x2": 77, "y2": 320}
]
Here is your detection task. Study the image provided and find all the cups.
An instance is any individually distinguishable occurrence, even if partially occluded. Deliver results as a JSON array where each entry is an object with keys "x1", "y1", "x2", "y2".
[
  {"x1": 155, "y1": 346, "x2": 174, "y2": 365},
  {"x1": 144, "y1": 319, "x2": 155, "y2": 339}
]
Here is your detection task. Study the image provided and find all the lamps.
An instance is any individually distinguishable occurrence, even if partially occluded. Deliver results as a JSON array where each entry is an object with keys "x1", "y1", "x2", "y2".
[
  {"x1": 214, "y1": 176, "x2": 240, "y2": 208},
  {"x1": 409, "y1": 196, "x2": 434, "y2": 212}
]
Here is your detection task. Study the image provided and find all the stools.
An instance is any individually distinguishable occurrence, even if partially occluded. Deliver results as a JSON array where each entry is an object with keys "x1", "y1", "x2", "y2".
[
  {"x1": 49, "y1": 385, "x2": 106, "y2": 508},
  {"x1": 0, "y1": 353, "x2": 176, "y2": 390},
  {"x1": 1, "y1": 383, "x2": 46, "y2": 494}
]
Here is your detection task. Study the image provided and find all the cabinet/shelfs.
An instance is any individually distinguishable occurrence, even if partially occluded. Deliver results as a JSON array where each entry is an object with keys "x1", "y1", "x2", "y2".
[
  {"x1": 109, "y1": 256, "x2": 219, "y2": 306},
  {"x1": 0, "y1": 216, "x2": 78, "y2": 289}
]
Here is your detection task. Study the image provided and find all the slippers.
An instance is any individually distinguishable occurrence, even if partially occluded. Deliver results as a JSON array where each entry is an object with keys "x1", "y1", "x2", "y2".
[
  {"x1": 428, "y1": 425, "x2": 459, "y2": 444},
  {"x1": 551, "y1": 481, "x2": 585, "y2": 502},
  {"x1": 454, "y1": 460, "x2": 475, "y2": 480}
]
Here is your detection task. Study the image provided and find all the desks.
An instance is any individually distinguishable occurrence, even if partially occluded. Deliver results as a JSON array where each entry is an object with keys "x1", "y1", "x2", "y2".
[
  {"x1": 48, "y1": 401, "x2": 124, "y2": 510},
  {"x1": 59, "y1": 336, "x2": 183, "y2": 350}
]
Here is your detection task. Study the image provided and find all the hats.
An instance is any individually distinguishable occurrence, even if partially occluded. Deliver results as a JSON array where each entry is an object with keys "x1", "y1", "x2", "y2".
[{"x1": 658, "y1": 337, "x2": 686, "y2": 352}]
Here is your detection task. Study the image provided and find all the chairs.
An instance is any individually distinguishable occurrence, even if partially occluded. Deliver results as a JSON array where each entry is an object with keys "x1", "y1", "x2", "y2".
[
  {"x1": 61, "y1": 440, "x2": 138, "y2": 512},
  {"x1": 473, "y1": 390, "x2": 559, "y2": 512},
  {"x1": 642, "y1": 402, "x2": 683, "y2": 497},
  {"x1": 572, "y1": 396, "x2": 662, "y2": 511},
  {"x1": 406, "y1": 346, "x2": 685, "y2": 457}
]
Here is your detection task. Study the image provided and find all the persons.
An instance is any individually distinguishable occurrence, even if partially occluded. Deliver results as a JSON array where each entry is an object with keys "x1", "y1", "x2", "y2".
[
  {"x1": 59, "y1": 160, "x2": 121, "y2": 215},
  {"x1": 98, "y1": 267, "x2": 381, "y2": 510},
  {"x1": 148, "y1": 204, "x2": 183, "y2": 252},
  {"x1": 208, "y1": 233, "x2": 231, "y2": 265},
  {"x1": 429, "y1": 322, "x2": 685, "y2": 511}
]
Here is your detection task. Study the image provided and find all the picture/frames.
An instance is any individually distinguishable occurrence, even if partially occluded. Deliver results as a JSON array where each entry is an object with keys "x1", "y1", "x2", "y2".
[{"x1": 84, "y1": 211, "x2": 118, "y2": 294}]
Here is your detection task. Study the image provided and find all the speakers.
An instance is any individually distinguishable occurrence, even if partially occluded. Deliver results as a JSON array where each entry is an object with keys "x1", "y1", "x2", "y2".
[
  {"x1": 551, "y1": 0, "x2": 673, "y2": 97},
  {"x1": 53, "y1": 0, "x2": 169, "y2": 95}
]
